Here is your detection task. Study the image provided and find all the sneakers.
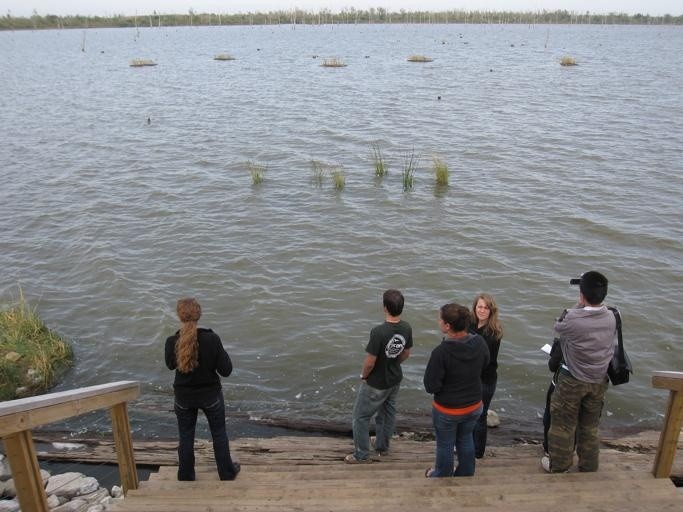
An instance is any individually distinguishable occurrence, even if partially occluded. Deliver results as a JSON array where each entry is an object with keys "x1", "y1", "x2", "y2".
[
  {"x1": 232, "y1": 461, "x2": 242, "y2": 479},
  {"x1": 542, "y1": 457, "x2": 551, "y2": 470}
]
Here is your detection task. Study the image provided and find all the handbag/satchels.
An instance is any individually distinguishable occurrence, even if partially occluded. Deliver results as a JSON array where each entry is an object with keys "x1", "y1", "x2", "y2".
[{"x1": 608, "y1": 306, "x2": 634, "y2": 385}]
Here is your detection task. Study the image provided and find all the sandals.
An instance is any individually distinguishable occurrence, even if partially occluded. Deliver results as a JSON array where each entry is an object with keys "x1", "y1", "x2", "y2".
[
  {"x1": 345, "y1": 454, "x2": 372, "y2": 464},
  {"x1": 425, "y1": 468, "x2": 435, "y2": 478}
]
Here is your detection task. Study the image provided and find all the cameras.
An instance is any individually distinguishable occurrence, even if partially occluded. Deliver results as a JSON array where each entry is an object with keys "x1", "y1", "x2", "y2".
[{"x1": 570, "y1": 279, "x2": 580, "y2": 284}]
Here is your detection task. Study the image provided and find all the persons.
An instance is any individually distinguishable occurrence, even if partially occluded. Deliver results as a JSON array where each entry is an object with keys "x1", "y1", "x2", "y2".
[
  {"x1": 541, "y1": 271, "x2": 621, "y2": 473},
  {"x1": 541, "y1": 333, "x2": 564, "y2": 457},
  {"x1": 424, "y1": 304, "x2": 490, "y2": 478},
  {"x1": 466, "y1": 293, "x2": 502, "y2": 461},
  {"x1": 343, "y1": 289, "x2": 413, "y2": 463},
  {"x1": 165, "y1": 298, "x2": 240, "y2": 482}
]
